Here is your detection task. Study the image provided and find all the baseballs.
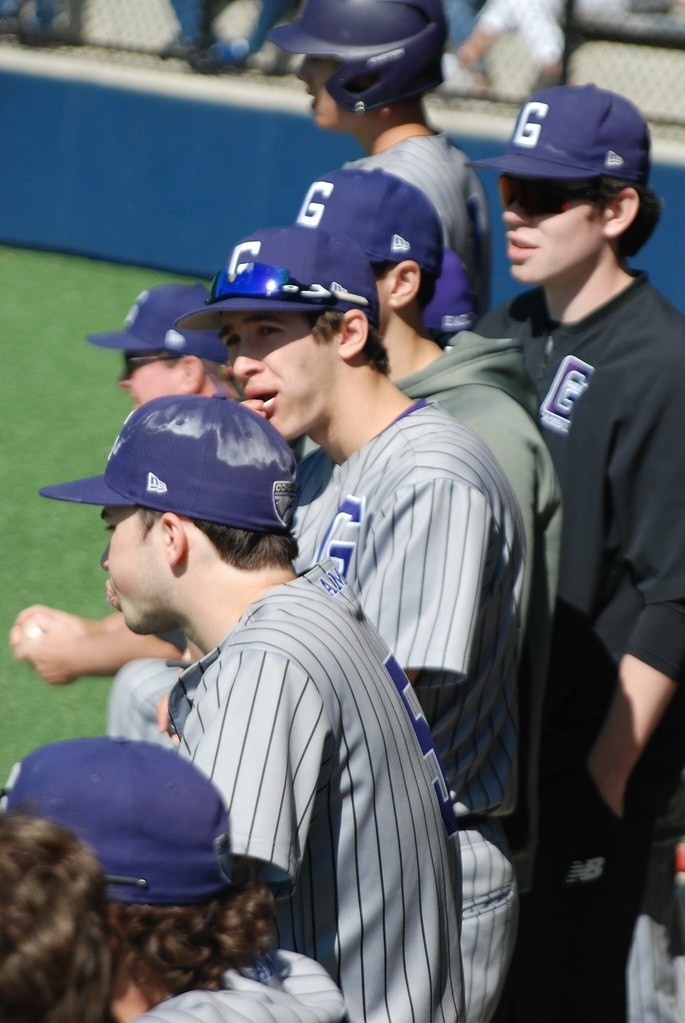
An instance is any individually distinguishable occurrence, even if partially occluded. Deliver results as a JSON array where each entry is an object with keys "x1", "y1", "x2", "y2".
[{"x1": 23, "y1": 616, "x2": 44, "y2": 641}]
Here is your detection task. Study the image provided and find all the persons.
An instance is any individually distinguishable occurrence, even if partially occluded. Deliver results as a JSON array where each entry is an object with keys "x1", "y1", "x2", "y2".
[
  {"x1": 38, "y1": 386, "x2": 469, "y2": 1023},
  {"x1": 0, "y1": 733, "x2": 352, "y2": 1023},
  {"x1": 158, "y1": 1, "x2": 300, "y2": 75},
  {"x1": 1, "y1": 800, "x2": 135, "y2": 1023},
  {"x1": 462, "y1": 81, "x2": 685, "y2": 1023},
  {"x1": 267, "y1": 0, "x2": 494, "y2": 316},
  {"x1": 9, "y1": 282, "x2": 240, "y2": 739},
  {"x1": 174, "y1": 214, "x2": 528, "y2": 1023},
  {"x1": 0, "y1": 1, "x2": 88, "y2": 47},
  {"x1": 440, "y1": 0, "x2": 568, "y2": 105},
  {"x1": 293, "y1": 169, "x2": 564, "y2": 898}
]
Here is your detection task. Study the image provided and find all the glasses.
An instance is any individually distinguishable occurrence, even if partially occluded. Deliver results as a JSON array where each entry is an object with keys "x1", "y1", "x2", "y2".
[
  {"x1": 501, "y1": 174, "x2": 609, "y2": 218},
  {"x1": 207, "y1": 263, "x2": 369, "y2": 307},
  {"x1": 124, "y1": 353, "x2": 186, "y2": 371}
]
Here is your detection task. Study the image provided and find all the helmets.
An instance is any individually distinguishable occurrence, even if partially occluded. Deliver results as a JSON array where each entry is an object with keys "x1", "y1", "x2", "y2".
[{"x1": 266, "y1": 1, "x2": 452, "y2": 114}]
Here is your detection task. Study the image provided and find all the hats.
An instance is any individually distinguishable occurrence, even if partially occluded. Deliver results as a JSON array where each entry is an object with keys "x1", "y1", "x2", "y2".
[
  {"x1": 464, "y1": 83, "x2": 650, "y2": 181},
  {"x1": 38, "y1": 394, "x2": 300, "y2": 529},
  {"x1": 294, "y1": 169, "x2": 444, "y2": 279},
  {"x1": 172, "y1": 226, "x2": 382, "y2": 329},
  {"x1": 87, "y1": 284, "x2": 229, "y2": 363},
  {"x1": 0, "y1": 736, "x2": 236, "y2": 905}
]
